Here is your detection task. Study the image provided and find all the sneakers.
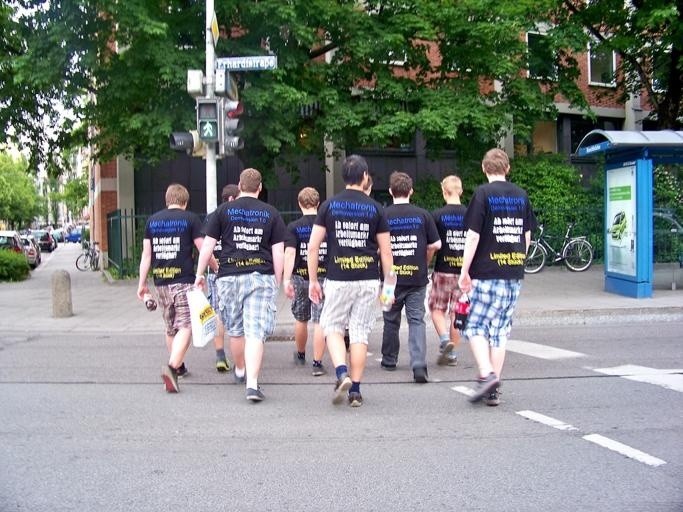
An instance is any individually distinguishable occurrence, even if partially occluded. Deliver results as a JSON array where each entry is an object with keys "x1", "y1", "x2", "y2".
[
  {"x1": 413, "y1": 367, "x2": 427, "y2": 383},
  {"x1": 467, "y1": 372, "x2": 500, "y2": 405},
  {"x1": 334, "y1": 374, "x2": 362, "y2": 406},
  {"x1": 437, "y1": 340, "x2": 457, "y2": 366},
  {"x1": 294, "y1": 353, "x2": 326, "y2": 376},
  {"x1": 381, "y1": 361, "x2": 396, "y2": 370},
  {"x1": 163, "y1": 363, "x2": 187, "y2": 393},
  {"x1": 216, "y1": 359, "x2": 264, "y2": 400}
]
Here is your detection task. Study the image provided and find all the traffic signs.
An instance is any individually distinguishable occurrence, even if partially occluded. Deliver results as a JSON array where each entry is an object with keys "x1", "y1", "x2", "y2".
[{"x1": 216, "y1": 55, "x2": 278, "y2": 71}]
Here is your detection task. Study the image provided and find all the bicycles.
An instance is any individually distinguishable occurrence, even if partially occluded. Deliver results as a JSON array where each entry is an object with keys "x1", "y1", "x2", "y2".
[
  {"x1": 522, "y1": 219, "x2": 593, "y2": 274},
  {"x1": 76, "y1": 239, "x2": 100, "y2": 271}
]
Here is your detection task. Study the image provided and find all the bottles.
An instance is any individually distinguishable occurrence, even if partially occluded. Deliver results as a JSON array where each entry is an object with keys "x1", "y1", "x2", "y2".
[
  {"x1": 453, "y1": 291, "x2": 471, "y2": 330},
  {"x1": 378, "y1": 270, "x2": 397, "y2": 312},
  {"x1": 142, "y1": 288, "x2": 157, "y2": 312}
]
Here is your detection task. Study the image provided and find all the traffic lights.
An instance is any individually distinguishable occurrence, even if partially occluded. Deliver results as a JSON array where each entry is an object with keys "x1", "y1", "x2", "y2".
[
  {"x1": 197, "y1": 99, "x2": 219, "y2": 139},
  {"x1": 221, "y1": 97, "x2": 244, "y2": 156},
  {"x1": 169, "y1": 130, "x2": 206, "y2": 157}
]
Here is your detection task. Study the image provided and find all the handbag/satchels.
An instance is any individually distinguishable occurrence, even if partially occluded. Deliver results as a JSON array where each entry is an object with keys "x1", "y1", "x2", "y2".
[{"x1": 187, "y1": 288, "x2": 217, "y2": 348}]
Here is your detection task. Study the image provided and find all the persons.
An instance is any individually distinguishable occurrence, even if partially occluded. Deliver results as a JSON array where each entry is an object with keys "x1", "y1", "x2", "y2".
[
  {"x1": 345, "y1": 175, "x2": 374, "y2": 352},
  {"x1": 202, "y1": 185, "x2": 241, "y2": 372},
  {"x1": 307, "y1": 155, "x2": 396, "y2": 406},
  {"x1": 457, "y1": 148, "x2": 539, "y2": 406},
  {"x1": 629, "y1": 215, "x2": 636, "y2": 253},
  {"x1": 138, "y1": 184, "x2": 219, "y2": 392},
  {"x1": 377, "y1": 170, "x2": 442, "y2": 383},
  {"x1": 195, "y1": 168, "x2": 290, "y2": 400},
  {"x1": 283, "y1": 187, "x2": 327, "y2": 375},
  {"x1": 428, "y1": 176, "x2": 467, "y2": 366}
]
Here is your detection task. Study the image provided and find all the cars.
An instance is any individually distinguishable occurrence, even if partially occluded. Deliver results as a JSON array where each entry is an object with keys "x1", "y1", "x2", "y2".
[
  {"x1": 65, "y1": 229, "x2": 81, "y2": 243},
  {"x1": 0, "y1": 228, "x2": 65, "y2": 269}
]
